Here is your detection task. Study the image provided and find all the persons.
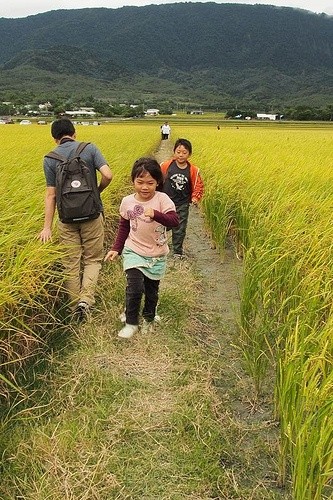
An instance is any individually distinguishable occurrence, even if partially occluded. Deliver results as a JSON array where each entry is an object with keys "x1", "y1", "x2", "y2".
[
  {"x1": 37, "y1": 118, "x2": 113, "y2": 321},
  {"x1": 160, "y1": 121, "x2": 171, "y2": 140},
  {"x1": 158, "y1": 139, "x2": 204, "y2": 258},
  {"x1": 104, "y1": 157, "x2": 179, "y2": 338}
]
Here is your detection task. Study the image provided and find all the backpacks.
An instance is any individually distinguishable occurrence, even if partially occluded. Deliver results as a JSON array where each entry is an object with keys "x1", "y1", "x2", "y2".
[{"x1": 44, "y1": 142, "x2": 101, "y2": 224}]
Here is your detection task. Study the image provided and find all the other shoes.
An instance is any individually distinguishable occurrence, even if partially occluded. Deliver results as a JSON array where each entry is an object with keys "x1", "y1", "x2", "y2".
[
  {"x1": 77, "y1": 302, "x2": 89, "y2": 323},
  {"x1": 120, "y1": 307, "x2": 127, "y2": 322},
  {"x1": 173, "y1": 254, "x2": 182, "y2": 260},
  {"x1": 154, "y1": 306, "x2": 161, "y2": 321}
]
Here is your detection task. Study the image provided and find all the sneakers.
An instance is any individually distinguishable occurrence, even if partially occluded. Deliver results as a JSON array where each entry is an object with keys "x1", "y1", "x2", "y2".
[
  {"x1": 141, "y1": 319, "x2": 154, "y2": 335},
  {"x1": 118, "y1": 323, "x2": 138, "y2": 339}
]
